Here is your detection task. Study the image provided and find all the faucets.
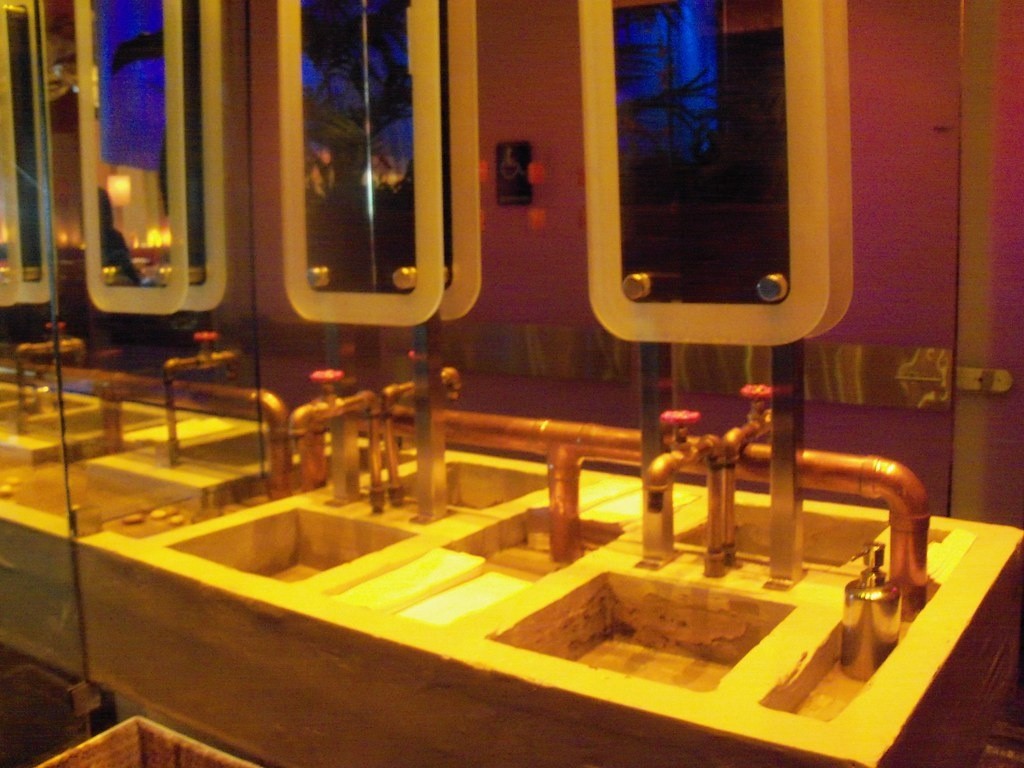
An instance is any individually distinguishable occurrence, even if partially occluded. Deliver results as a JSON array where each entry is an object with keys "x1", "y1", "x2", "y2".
[
  {"x1": 162, "y1": 328, "x2": 242, "y2": 448},
  {"x1": 642, "y1": 410, "x2": 732, "y2": 576},
  {"x1": 722, "y1": 383, "x2": 783, "y2": 558},
  {"x1": 17, "y1": 319, "x2": 87, "y2": 415},
  {"x1": 385, "y1": 350, "x2": 464, "y2": 508}
]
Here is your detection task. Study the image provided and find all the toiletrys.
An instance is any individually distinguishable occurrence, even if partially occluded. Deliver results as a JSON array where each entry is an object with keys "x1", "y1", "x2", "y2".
[{"x1": 839, "y1": 539, "x2": 904, "y2": 680}]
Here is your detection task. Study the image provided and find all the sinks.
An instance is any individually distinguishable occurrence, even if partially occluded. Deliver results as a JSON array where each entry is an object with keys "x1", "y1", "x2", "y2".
[
  {"x1": 182, "y1": 427, "x2": 314, "y2": 473},
  {"x1": 483, "y1": 564, "x2": 801, "y2": 705},
  {"x1": 166, "y1": 502, "x2": 423, "y2": 588},
  {"x1": 42, "y1": 403, "x2": 172, "y2": 441},
  {"x1": 381, "y1": 456, "x2": 558, "y2": 513},
  {"x1": 672, "y1": 499, "x2": 892, "y2": 578},
  {"x1": 288, "y1": 366, "x2": 387, "y2": 514}
]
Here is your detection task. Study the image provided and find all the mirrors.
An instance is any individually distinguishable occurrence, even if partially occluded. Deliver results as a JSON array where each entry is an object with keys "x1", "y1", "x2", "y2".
[
  {"x1": 274, "y1": 0, "x2": 446, "y2": 324},
  {"x1": 243, "y1": 0, "x2": 963, "y2": 521},
  {"x1": 181, "y1": 0, "x2": 225, "y2": 315},
  {"x1": 577, "y1": 0, "x2": 832, "y2": 346},
  {"x1": 299, "y1": 1, "x2": 377, "y2": 291},
  {"x1": 439, "y1": 0, "x2": 483, "y2": 321},
  {"x1": 2, "y1": 0, "x2": 54, "y2": 304},
  {"x1": 2, "y1": 1, "x2": 97, "y2": 765},
  {"x1": 41, "y1": 1, "x2": 264, "y2": 532},
  {"x1": 73, "y1": 0, "x2": 188, "y2": 313}
]
